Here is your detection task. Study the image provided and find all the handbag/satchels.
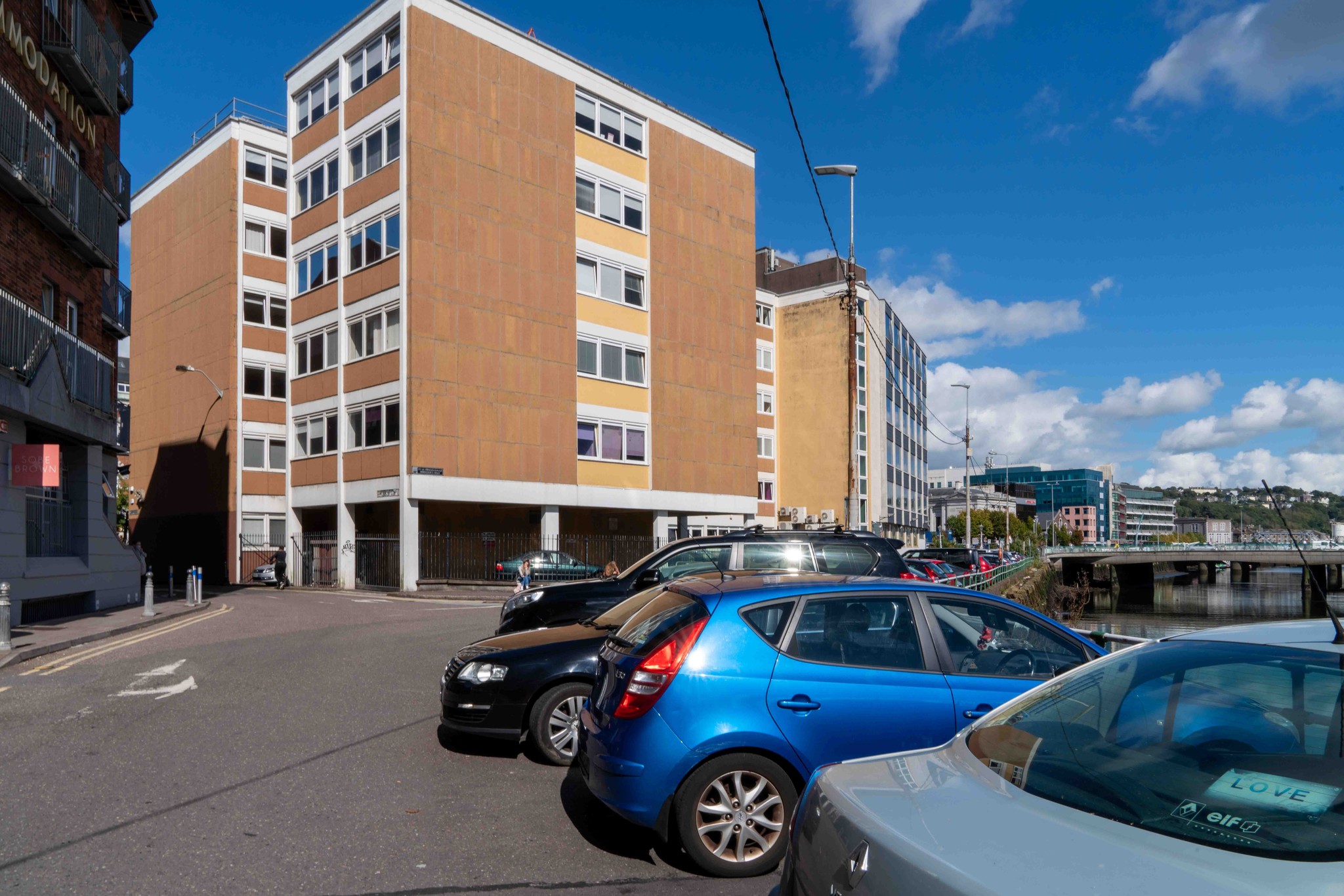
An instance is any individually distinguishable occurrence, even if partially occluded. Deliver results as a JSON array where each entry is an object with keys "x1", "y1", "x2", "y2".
[{"x1": 516, "y1": 577, "x2": 520, "y2": 582}]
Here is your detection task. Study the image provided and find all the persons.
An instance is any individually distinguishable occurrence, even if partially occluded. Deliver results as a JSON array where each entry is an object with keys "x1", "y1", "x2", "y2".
[
  {"x1": 1330, "y1": 539, "x2": 1332, "y2": 550},
  {"x1": 519, "y1": 559, "x2": 532, "y2": 591},
  {"x1": 1208, "y1": 539, "x2": 1220, "y2": 549},
  {"x1": 132, "y1": 541, "x2": 147, "y2": 599},
  {"x1": 270, "y1": 546, "x2": 287, "y2": 590},
  {"x1": 1081, "y1": 543, "x2": 1087, "y2": 552},
  {"x1": 1255, "y1": 539, "x2": 1259, "y2": 550},
  {"x1": 1140, "y1": 542, "x2": 1143, "y2": 550},
  {"x1": 1068, "y1": 542, "x2": 1074, "y2": 553},
  {"x1": 603, "y1": 561, "x2": 620, "y2": 578},
  {"x1": 1093, "y1": 542, "x2": 1097, "y2": 552},
  {"x1": 1303, "y1": 539, "x2": 1309, "y2": 550},
  {"x1": 1114, "y1": 542, "x2": 1119, "y2": 552},
  {"x1": 1242, "y1": 535, "x2": 1247, "y2": 549},
  {"x1": 1289, "y1": 542, "x2": 1292, "y2": 550},
  {"x1": 1056, "y1": 543, "x2": 1060, "y2": 554}
]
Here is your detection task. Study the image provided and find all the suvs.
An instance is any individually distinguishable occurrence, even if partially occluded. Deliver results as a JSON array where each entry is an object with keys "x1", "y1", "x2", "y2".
[
  {"x1": 495, "y1": 521, "x2": 915, "y2": 636},
  {"x1": 1312, "y1": 541, "x2": 1344, "y2": 550}
]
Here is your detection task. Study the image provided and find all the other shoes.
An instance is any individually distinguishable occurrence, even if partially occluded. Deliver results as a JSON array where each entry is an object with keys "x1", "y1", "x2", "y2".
[
  {"x1": 276, "y1": 586, "x2": 279, "y2": 589},
  {"x1": 279, "y1": 582, "x2": 285, "y2": 589}
]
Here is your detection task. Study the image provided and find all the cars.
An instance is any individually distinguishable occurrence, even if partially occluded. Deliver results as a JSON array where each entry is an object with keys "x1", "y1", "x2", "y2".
[
  {"x1": 495, "y1": 550, "x2": 605, "y2": 581},
  {"x1": 769, "y1": 615, "x2": 1344, "y2": 896},
  {"x1": 252, "y1": 562, "x2": 278, "y2": 584},
  {"x1": 1189, "y1": 543, "x2": 1215, "y2": 550},
  {"x1": 900, "y1": 547, "x2": 1028, "y2": 591},
  {"x1": 575, "y1": 566, "x2": 1308, "y2": 881},
  {"x1": 436, "y1": 576, "x2": 671, "y2": 769}
]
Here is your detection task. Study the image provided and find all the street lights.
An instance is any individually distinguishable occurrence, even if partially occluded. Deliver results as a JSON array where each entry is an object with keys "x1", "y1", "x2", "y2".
[
  {"x1": 1046, "y1": 483, "x2": 1060, "y2": 548},
  {"x1": 989, "y1": 452, "x2": 1010, "y2": 551},
  {"x1": 1157, "y1": 519, "x2": 1160, "y2": 551},
  {"x1": 1176, "y1": 524, "x2": 1179, "y2": 550},
  {"x1": 1239, "y1": 506, "x2": 1243, "y2": 543},
  {"x1": 1134, "y1": 517, "x2": 1138, "y2": 551},
  {"x1": 814, "y1": 163, "x2": 862, "y2": 529},
  {"x1": 937, "y1": 525, "x2": 942, "y2": 548},
  {"x1": 979, "y1": 524, "x2": 983, "y2": 549},
  {"x1": 951, "y1": 383, "x2": 972, "y2": 550}
]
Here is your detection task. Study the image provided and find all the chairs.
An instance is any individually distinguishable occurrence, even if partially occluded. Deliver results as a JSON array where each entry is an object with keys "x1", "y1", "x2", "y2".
[
  {"x1": 838, "y1": 602, "x2": 877, "y2": 667},
  {"x1": 883, "y1": 607, "x2": 925, "y2": 670}
]
